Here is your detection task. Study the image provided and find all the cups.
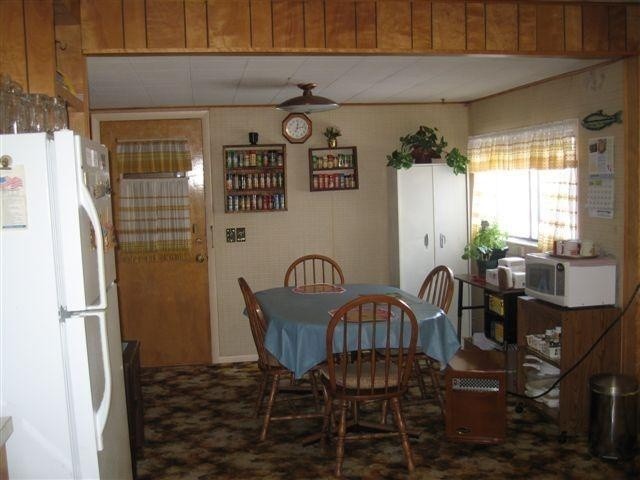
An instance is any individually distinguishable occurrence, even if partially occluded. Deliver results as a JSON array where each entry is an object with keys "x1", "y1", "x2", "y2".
[{"x1": 0, "y1": 73, "x2": 68, "y2": 134}]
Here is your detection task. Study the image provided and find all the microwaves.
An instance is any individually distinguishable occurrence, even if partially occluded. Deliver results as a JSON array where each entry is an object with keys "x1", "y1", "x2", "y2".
[{"x1": 523, "y1": 253, "x2": 617, "y2": 309}]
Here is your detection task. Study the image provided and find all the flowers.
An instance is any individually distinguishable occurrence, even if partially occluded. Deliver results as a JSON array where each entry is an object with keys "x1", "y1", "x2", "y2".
[{"x1": 323, "y1": 126, "x2": 341, "y2": 138}]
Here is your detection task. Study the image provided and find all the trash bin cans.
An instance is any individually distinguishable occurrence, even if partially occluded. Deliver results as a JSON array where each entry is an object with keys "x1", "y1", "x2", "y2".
[{"x1": 588, "y1": 372, "x2": 639, "y2": 465}]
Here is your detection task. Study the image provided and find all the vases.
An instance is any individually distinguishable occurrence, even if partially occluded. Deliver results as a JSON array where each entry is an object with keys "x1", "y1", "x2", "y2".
[{"x1": 328, "y1": 139, "x2": 338, "y2": 148}]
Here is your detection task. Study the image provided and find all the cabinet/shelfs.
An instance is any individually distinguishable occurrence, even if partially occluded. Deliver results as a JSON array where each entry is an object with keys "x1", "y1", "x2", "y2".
[
  {"x1": 384, "y1": 162, "x2": 472, "y2": 339},
  {"x1": 222, "y1": 144, "x2": 288, "y2": 213},
  {"x1": 308, "y1": 146, "x2": 360, "y2": 191},
  {"x1": 516, "y1": 295, "x2": 621, "y2": 426},
  {"x1": 454, "y1": 272, "x2": 525, "y2": 397}
]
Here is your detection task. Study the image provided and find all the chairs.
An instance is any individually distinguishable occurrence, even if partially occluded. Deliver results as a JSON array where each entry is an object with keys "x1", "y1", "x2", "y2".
[
  {"x1": 320, "y1": 293, "x2": 419, "y2": 476},
  {"x1": 283, "y1": 254, "x2": 345, "y2": 386},
  {"x1": 237, "y1": 275, "x2": 339, "y2": 440},
  {"x1": 375, "y1": 265, "x2": 455, "y2": 422}
]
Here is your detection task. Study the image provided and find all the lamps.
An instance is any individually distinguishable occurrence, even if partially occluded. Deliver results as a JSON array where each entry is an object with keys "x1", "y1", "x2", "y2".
[{"x1": 274, "y1": 82, "x2": 338, "y2": 114}]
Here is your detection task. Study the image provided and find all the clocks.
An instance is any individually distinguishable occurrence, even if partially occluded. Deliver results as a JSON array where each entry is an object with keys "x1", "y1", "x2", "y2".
[{"x1": 281, "y1": 113, "x2": 312, "y2": 144}]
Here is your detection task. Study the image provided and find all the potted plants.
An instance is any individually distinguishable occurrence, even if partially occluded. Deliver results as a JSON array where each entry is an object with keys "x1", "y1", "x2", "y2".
[
  {"x1": 461, "y1": 221, "x2": 510, "y2": 275},
  {"x1": 388, "y1": 127, "x2": 471, "y2": 177}
]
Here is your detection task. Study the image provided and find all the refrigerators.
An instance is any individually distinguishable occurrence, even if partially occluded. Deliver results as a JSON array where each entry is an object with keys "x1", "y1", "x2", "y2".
[{"x1": 0, "y1": 128, "x2": 134, "y2": 480}]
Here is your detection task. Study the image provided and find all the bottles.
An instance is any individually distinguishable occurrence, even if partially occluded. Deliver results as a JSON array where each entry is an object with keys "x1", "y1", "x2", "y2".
[
  {"x1": 280, "y1": 194, "x2": 285, "y2": 209},
  {"x1": 340, "y1": 175, "x2": 345, "y2": 189},
  {"x1": 264, "y1": 151, "x2": 268, "y2": 167},
  {"x1": 244, "y1": 151, "x2": 249, "y2": 168},
  {"x1": 318, "y1": 152, "x2": 323, "y2": 171},
  {"x1": 335, "y1": 175, "x2": 340, "y2": 189},
  {"x1": 313, "y1": 174, "x2": 318, "y2": 189},
  {"x1": 338, "y1": 154, "x2": 344, "y2": 168},
  {"x1": 348, "y1": 173, "x2": 351, "y2": 189},
  {"x1": 260, "y1": 173, "x2": 265, "y2": 188},
  {"x1": 226, "y1": 174, "x2": 233, "y2": 190},
  {"x1": 319, "y1": 175, "x2": 324, "y2": 190},
  {"x1": 345, "y1": 176, "x2": 349, "y2": 189},
  {"x1": 241, "y1": 175, "x2": 246, "y2": 190},
  {"x1": 312, "y1": 152, "x2": 317, "y2": 171},
  {"x1": 327, "y1": 155, "x2": 334, "y2": 170},
  {"x1": 248, "y1": 174, "x2": 252, "y2": 189},
  {"x1": 272, "y1": 173, "x2": 277, "y2": 188},
  {"x1": 266, "y1": 173, "x2": 270, "y2": 189},
  {"x1": 228, "y1": 195, "x2": 233, "y2": 211},
  {"x1": 250, "y1": 151, "x2": 256, "y2": 167},
  {"x1": 329, "y1": 175, "x2": 335, "y2": 189},
  {"x1": 274, "y1": 195, "x2": 279, "y2": 210},
  {"x1": 324, "y1": 153, "x2": 328, "y2": 170},
  {"x1": 351, "y1": 174, "x2": 356, "y2": 188},
  {"x1": 253, "y1": 174, "x2": 258, "y2": 189},
  {"x1": 234, "y1": 174, "x2": 239, "y2": 190},
  {"x1": 263, "y1": 195, "x2": 268, "y2": 210},
  {"x1": 233, "y1": 151, "x2": 238, "y2": 168},
  {"x1": 245, "y1": 195, "x2": 250, "y2": 210},
  {"x1": 349, "y1": 155, "x2": 354, "y2": 168},
  {"x1": 251, "y1": 194, "x2": 256, "y2": 210},
  {"x1": 257, "y1": 195, "x2": 262, "y2": 210},
  {"x1": 240, "y1": 196, "x2": 245, "y2": 210},
  {"x1": 239, "y1": 151, "x2": 243, "y2": 168},
  {"x1": 270, "y1": 151, "x2": 275, "y2": 166},
  {"x1": 268, "y1": 195, "x2": 273, "y2": 210},
  {"x1": 227, "y1": 151, "x2": 232, "y2": 168},
  {"x1": 334, "y1": 156, "x2": 338, "y2": 170},
  {"x1": 324, "y1": 174, "x2": 329, "y2": 189},
  {"x1": 278, "y1": 151, "x2": 283, "y2": 167},
  {"x1": 234, "y1": 195, "x2": 238, "y2": 211},
  {"x1": 257, "y1": 152, "x2": 262, "y2": 167},
  {"x1": 278, "y1": 172, "x2": 283, "y2": 188},
  {"x1": 345, "y1": 155, "x2": 349, "y2": 168}
]
